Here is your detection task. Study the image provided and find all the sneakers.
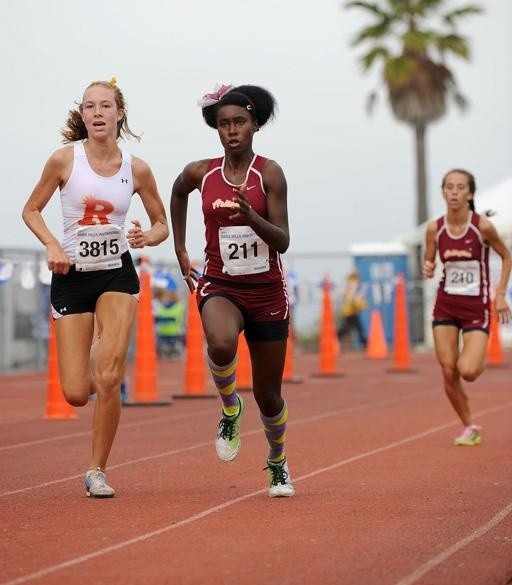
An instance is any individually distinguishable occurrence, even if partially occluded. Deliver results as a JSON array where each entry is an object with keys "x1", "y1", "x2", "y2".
[
  {"x1": 215, "y1": 395, "x2": 243, "y2": 462},
  {"x1": 85, "y1": 467, "x2": 115, "y2": 498},
  {"x1": 89, "y1": 392, "x2": 98, "y2": 400},
  {"x1": 262, "y1": 457, "x2": 295, "y2": 498},
  {"x1": 454, "y1": 425, "x2": 481, "y2": 447}
]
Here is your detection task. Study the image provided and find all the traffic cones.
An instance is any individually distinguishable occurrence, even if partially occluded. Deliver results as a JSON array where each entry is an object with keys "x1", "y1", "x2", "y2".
[
  {"x1": 176, "y1": 280, "x2": 218, "y2": 400},
  {"x1": 237, "y1": 328, "x2": 260, "y2": 392},
  {"x1": 125, "y1": 265, "x2": 168, "y2": 403},
  {"x1": 389, "y1": 271, "x2": 422, "y2": 377},
  {"x1": 365, "y1": 308, "x2": 391, "y2": 359},
  {"x1": 317, "y1": 272, "x2": 343, "y2": 376},
  {"x1": 484, "y1": 297, "x2": 507, "y2": 370},
  {"x1": 275, "y1": 304, "x2": 304, "y2": 383},
  {"x1": 40, "y1": 300, "x2": 80, "y2": 421}
]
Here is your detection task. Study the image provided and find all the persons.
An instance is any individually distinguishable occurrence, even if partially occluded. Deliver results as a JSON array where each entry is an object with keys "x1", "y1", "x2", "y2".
[
  {"x1": 23, "y1": 82, "x2": 168, "y2": 496},
  {"x1": 424, "y1": 170, "x2": 512, "y2": 447},
  {"x1": 171, "y1": 87, "x2": 294, "y2": 499}
]
